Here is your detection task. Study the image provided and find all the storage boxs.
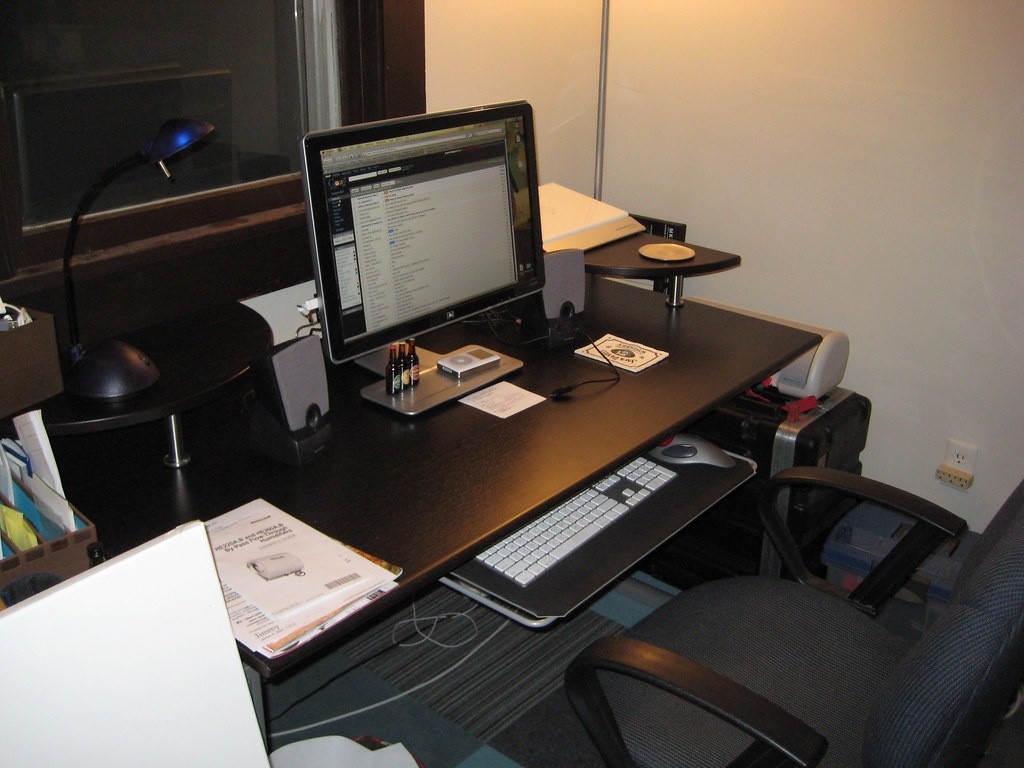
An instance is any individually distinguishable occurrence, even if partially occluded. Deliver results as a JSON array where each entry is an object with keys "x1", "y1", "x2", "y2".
[
  {"x1": 0, "y1": 301, "x2": 64, "y2": 420},
  {"x1": 822, "y1": 499, "x2": 981, "y2": 642},
  {"x1": 0, "y1": 473, "x2": 98, "y2": 593}
]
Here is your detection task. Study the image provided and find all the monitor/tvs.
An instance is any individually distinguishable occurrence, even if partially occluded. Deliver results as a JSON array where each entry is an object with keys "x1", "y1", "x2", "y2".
[{"x1": 304, "y1": 99, "x2": 546, "y2": 415}]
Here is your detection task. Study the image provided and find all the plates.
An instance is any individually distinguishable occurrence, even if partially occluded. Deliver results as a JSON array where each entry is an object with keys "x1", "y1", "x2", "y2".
[{"x1": 639, "y1": 244, "x2": 696, "y2": 260}]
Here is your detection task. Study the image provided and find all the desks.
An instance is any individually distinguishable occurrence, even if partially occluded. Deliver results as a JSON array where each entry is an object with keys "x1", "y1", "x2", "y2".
[{"x1": 0, "y1": 230, "x2": 823, "y2": 746}]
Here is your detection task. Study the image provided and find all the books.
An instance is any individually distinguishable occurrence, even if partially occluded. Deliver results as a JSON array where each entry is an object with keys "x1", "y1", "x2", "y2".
[{"x1": 0, "y1": 437, "x2": 32, "y2": 507}]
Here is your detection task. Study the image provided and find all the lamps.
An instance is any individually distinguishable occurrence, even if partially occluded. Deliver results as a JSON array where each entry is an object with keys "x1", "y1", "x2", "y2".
[{"x1": 57, "y1": 117, "x2": 222, "y2": 402}]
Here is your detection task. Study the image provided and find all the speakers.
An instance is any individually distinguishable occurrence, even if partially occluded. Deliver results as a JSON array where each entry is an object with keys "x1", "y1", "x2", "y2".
[
  {"x1": 521, "y1": 248, "x2": 586, "y2": 350},
  {"x1": 248, "y1": 334, "x2": 333, "y2": 468}
]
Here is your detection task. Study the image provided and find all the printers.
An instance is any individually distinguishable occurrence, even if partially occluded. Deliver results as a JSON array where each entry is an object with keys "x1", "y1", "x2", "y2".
[{"x1": 688, "y1": 297, "x2": 849, "y2": 421}]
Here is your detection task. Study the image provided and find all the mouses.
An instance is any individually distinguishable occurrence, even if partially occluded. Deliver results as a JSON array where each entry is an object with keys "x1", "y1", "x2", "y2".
[{"x1": 649, "y1": 434, "x2": 736, "y2": 471}]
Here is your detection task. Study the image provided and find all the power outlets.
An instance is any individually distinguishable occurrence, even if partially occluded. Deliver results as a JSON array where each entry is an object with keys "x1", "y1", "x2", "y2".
[{"x1": 934, "y1": 437, "x2": 979, "y2": 490}]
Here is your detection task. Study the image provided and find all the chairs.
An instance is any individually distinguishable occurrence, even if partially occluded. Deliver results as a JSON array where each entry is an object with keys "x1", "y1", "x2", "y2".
[{"x1": 564, "y1": 468, "x2": 1024, "y2": 768}]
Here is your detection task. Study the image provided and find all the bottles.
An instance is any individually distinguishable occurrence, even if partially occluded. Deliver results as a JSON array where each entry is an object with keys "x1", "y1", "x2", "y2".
[
  {"x1": 397, "y1": 343, "x2": 410, "y2": 391},
  {"x1": 409, "y1": 338, "x2": 419, "y2": 385},
  {"x1": 386, "y1": 344, "x2": 401, "y2": 395},
  {"x1": 87, "y1": 542, "x2": 110, "y2": 568}
]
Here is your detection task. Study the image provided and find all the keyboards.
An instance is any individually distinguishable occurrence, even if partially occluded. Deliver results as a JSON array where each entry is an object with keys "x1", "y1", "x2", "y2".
[{"x1": 475, "y1": 456, "x2": 679, "y2": 588}]
